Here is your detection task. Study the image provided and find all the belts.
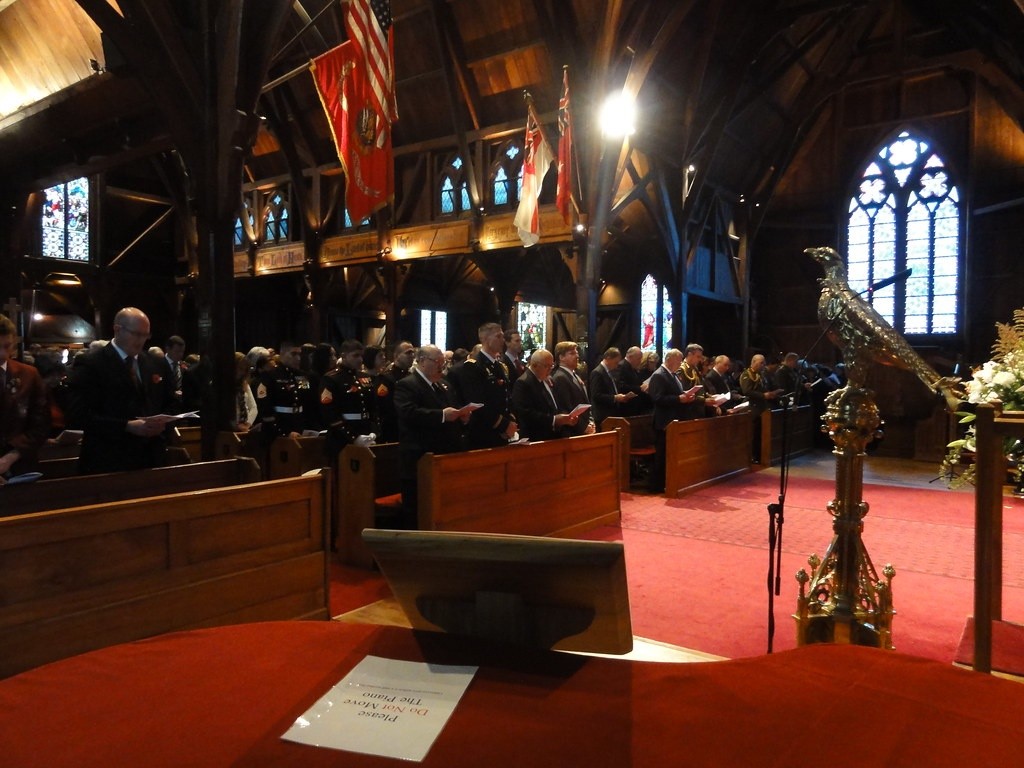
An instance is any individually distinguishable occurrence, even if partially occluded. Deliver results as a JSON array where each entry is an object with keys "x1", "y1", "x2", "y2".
[
  {"x1": 275, "y1": 406, "x2": 304, "y2": 414},
  {"x1": 341, "y1": 412, "x2": 370, "y2": 420}
]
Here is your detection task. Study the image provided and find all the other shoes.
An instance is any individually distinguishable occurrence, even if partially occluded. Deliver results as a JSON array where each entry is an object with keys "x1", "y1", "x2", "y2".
[{"x1": 751, "y1": 458, "x2": 760, "y2": 464}]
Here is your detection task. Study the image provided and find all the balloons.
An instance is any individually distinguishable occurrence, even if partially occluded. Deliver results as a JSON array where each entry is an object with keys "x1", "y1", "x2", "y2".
[{"x1": 667, "y1": 312, "x2": 672, "y2": 320}]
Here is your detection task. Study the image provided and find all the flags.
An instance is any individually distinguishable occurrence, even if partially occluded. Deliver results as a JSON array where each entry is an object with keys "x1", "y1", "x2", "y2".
[
  {"x1": 556, "y1": 74, "x2": 573, "y2": 225},
  {"x1": 513, "y1": 108, "x2": 553, "y2": 248},
  {"x1": 310, "y1": 0, "x2": 399, "y2": 224}
]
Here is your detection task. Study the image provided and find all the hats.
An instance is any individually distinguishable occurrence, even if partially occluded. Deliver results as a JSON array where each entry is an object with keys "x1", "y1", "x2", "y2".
[{"x1": 246, "y1": 345, "x2": 272, "y2": 368}]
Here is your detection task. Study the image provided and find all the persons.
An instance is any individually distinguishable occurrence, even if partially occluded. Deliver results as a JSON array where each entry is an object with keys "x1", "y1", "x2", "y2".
[{"x1": 0, "y1": 308, "x2": 845, "y2": 572}]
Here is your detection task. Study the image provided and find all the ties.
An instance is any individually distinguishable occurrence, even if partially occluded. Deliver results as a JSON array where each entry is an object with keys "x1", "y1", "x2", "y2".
[
  {"x1": 574, "y1": 373, "x2": 586, "y2": 395},
  {"x1": 494, "y1": 361, "x2": 502, "y2": 379},
  {"x1": 0, "y1": 367, "x2": 4, "y2": 392},
  {"x1": 125, "y1": 356, "x2": 140, "y2": 383},
  {"x1": 671, "y1": 373, "x2": 684, "y2": 391},
  {"x1": 540, "y1": 381, "x2": 556, "y2": 409},
  {"x1": 432, "y1": 383, "x2": 442, "y2": 392}
]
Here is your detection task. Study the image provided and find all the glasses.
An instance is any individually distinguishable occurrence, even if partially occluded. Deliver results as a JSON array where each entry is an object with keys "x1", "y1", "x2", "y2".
[
  {"x1": 425, "y1": 356, "x2": 444, "y2": 367},
  {"x1": 121, "y1": 326, "x2": 151, "y2": 340}
]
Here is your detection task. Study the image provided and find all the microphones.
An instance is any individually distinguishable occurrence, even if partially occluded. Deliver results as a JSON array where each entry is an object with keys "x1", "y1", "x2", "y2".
[{"x1": 870, "y1": 268, "x2": 912, "y2": 292}]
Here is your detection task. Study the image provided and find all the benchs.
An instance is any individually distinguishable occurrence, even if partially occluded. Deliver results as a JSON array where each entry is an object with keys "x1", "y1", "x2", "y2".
[
  {"x1": 600, "y1": 415, "x2": 661, "y2": 489},
  {"x1": 1, "y1": 374, "x2": 433, "y2": 569}
]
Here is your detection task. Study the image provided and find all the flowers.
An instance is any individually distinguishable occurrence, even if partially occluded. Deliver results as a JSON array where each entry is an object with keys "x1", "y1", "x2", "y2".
[{"x1": 940, "y1": 303, "x2": 1024, "y2": 493}]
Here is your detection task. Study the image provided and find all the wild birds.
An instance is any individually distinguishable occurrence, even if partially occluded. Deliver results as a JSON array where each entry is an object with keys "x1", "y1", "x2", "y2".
[{"x1": 802, "y1": 244, "x2": 960, "y2": 412}]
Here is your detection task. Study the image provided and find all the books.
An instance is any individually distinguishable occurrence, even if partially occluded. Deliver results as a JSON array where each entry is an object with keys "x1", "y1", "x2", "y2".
[
  {"x1": 303, "y1": 430, "x2": 327, "y2": 436},
  {"x1": 459, "y1": 403, "x2": 484, "y2": 413},
  {"x1": 570, "y1": 404, "x2": 591, "y2": 418},
  {"x1": 687, "y1": 386, "x2": 703, "y2": 397},
  {"x1": 810, "y1": 378, "x2": 822, "y2": 388},
  {"x1": 733, "y1": 401, "x2": 749, "y2": 410},
  {"x1": 774, "y1": 389, "x2": 784, "y2": 394},
  {"x1": 56, "y1": 430, "x2": 84, "y2": 442},
  {"x1": 625, "y1": 391, "x2": 638, "y2": 398},
  {"x1": 9, "y1": 472, "x2": 42, "y2": 483},
  {"x1": 137, "y1": 410, "x2": 200, "y2": 422},
  {"x1": 643, "y1": 378, "x2": 651, "y2": 391},
  {"x1": 713, "y1": 392, "x2": 730, "y2": 407}
]
